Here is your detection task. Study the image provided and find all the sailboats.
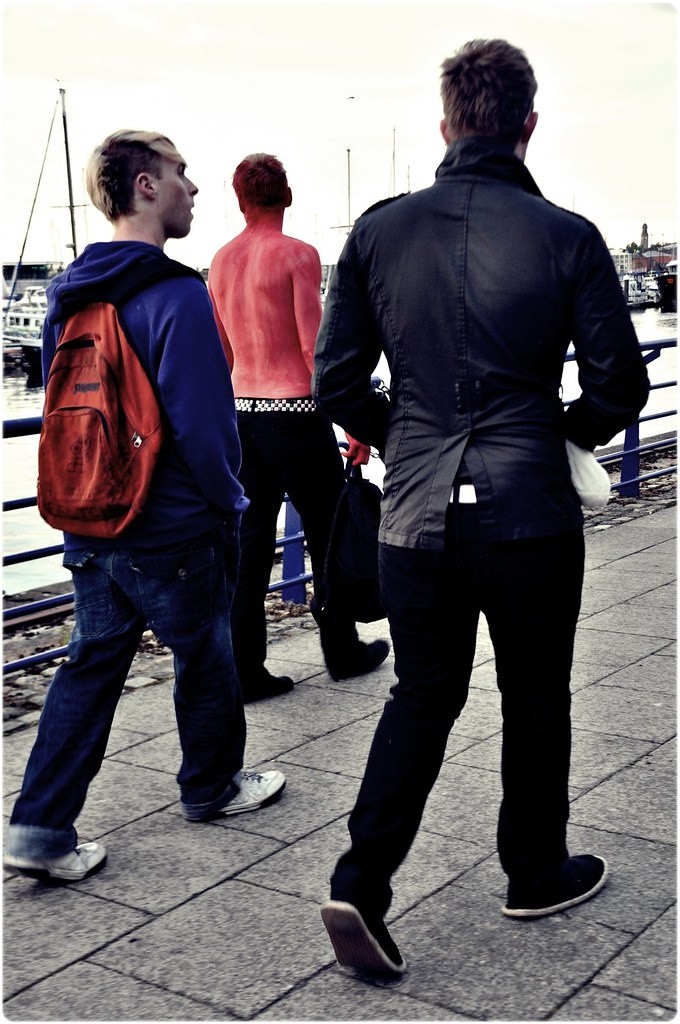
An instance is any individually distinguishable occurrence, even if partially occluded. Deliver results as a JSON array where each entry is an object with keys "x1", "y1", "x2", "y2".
[{"x1": 3, "y1": 88, "x2": 83, "y2": 346}]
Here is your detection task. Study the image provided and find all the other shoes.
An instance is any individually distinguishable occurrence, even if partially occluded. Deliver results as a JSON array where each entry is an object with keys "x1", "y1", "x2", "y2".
[
  {"x1": 322, "y1": 901, "x2": 406, "y2": 973},
  {"x1": 242, "y1": 677, "x2": 293, "y2": 703},
  {"x1": 329, "y1": 640, "x2": 389, "y2": 682},
  {"x1": 501, "y1": 855, "x2": 607, "y2": 917}
]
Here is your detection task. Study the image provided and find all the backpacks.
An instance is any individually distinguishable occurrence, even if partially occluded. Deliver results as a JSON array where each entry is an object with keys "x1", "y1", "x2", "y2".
[
  {"x1": 320, "y1": 457, "x2": 394, "y2": 623},
  {"x1": 38, "y1": 262, "x2": 205, "y2": 539}
]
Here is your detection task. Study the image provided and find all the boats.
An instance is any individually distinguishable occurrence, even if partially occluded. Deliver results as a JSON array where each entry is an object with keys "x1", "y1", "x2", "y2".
[{"x1": 654, "y1": 258, "x2": 677, "y2": 314}]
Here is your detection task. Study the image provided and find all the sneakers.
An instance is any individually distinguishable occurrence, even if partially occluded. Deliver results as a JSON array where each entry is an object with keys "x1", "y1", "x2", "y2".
[
  {"x1": 183, "y1": 770, "x2": 286, "y2": 820},
  {"x1": 2, "y1": 842, "x2": 107, "y2": 883}
]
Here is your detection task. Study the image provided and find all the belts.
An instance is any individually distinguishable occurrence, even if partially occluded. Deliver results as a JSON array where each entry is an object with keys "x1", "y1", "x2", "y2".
[
  {"x1": 448, "y1": 483, "x2": 477, "y2": 504},
  {"x1": 235, "y1": 399, "x2": 315, "y2": 412}
]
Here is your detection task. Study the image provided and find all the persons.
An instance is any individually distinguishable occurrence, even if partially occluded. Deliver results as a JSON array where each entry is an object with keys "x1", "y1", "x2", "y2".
[
  {"x1": 207, "y1": 150, "x2": 390, "y2": 701},
  {"x1": 312, "y1": 40, "x2": 652, "y2": 978},
  {"x1": 2, "y1": 131, "x2": 290, "y2": 883}
]
